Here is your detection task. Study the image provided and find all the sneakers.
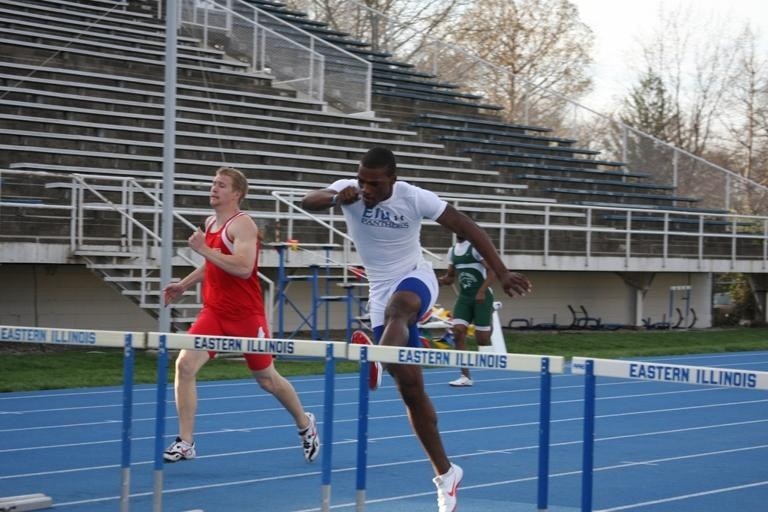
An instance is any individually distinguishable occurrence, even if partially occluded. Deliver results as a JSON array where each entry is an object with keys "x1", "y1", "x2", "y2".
[
  {"x1": 296, "y1": 411, "x2": 320, "y2": 462},
  {"x1": 447, "y1": 374, "x2": 475, "y2": 386},
  {"x1": 163, "y1": 438, "x2": 197, "y2": 462},
  {"x1": 351, "y1": 329, "x2": 383, "y2": 390},
  {"x1": 432, "y1": 461, "x2": 464, "y2": 512}
]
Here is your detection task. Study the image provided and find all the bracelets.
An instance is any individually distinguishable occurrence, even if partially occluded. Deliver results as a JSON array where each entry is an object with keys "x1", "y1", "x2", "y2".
[{"x1": 328, "y1": 193, "x2": 339, "y2": 206}]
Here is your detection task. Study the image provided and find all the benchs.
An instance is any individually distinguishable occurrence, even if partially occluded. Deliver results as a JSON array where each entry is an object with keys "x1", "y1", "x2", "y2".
[{"x1": 2, "y1": 2, "x2": 765, "y2": 256}]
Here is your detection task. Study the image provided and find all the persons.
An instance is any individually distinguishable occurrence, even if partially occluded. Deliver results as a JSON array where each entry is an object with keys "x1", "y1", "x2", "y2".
[
  {"x1": 436, "y1": 211, "x2": 504, "y2": 389},
  {"x1": 157, "y1": 168, "x2": 320, "y2": 464},
  {"x1": 302, "y1": 147, "x2": 533, "y2": 511}
]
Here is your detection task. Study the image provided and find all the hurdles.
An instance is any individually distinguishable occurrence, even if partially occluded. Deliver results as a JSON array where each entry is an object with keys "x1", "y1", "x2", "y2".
[
  {"x1": 145, "y1": 333, "x2": 347, "y2": 512},
  {"x1": 345, "y1": 342, "x2": 563, "y2": 512},
  {"x1": 0, "y1": 324, "x2": 145, "y2": 512},
  {"x1": 568, "y1": 354, "x2": 768, "y2": 512}
]
